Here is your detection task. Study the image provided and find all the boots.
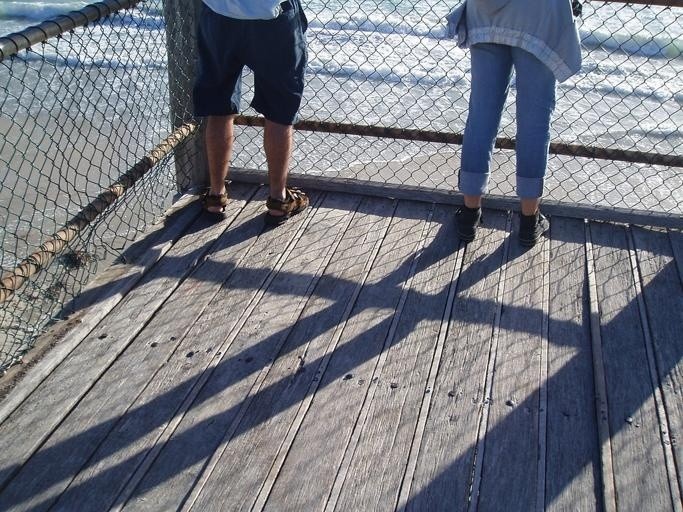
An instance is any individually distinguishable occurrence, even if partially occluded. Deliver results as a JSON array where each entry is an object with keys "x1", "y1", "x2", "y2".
[
  {"x1": 453, "y1": 208, "x2": 482, "y2": 239},
  {"x1": 519, "y1": 210, "x2": 550, "y2": 247}
]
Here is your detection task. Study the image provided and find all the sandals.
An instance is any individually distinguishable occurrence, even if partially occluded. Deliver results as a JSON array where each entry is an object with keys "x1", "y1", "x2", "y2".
[
  {"x1": 265, "y1": 187, "x2": 310, "y2": 226},
  {"x1": 198, "y1": 190, "x2": 228, "y2": 221}
]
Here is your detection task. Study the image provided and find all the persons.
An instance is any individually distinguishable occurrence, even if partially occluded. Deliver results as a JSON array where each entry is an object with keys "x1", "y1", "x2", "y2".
[
  {"x1": 190, "y1": 0, "x2": 310, "y2": 227},
  {"x1": 447, "y1": 0, "x2": 582, "y2": 248}
]
